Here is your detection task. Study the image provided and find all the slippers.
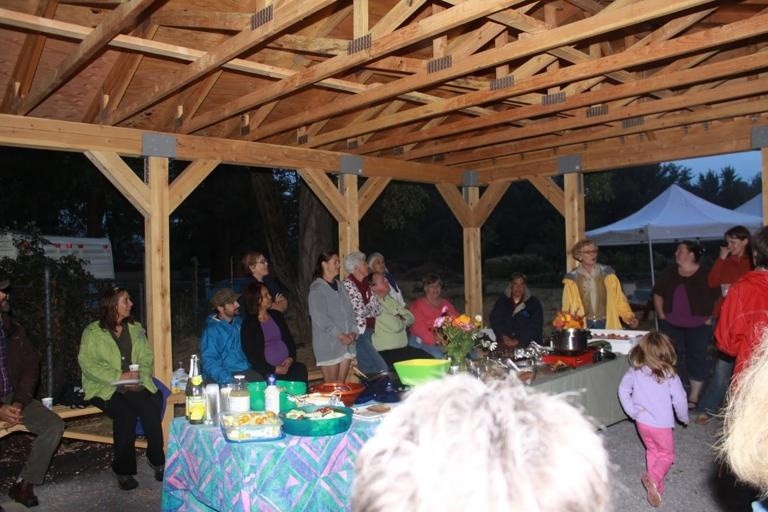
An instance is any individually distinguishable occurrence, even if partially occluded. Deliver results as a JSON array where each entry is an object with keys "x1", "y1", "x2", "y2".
[{"x1": 688, "y1": 401, "x2": 698, "y2": 410}]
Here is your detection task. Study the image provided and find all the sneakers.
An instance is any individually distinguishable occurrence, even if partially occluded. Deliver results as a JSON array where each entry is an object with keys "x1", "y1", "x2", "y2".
[
  {"x1": 111, "y1": 466, "x2": 138, "y2": 490},
  {"x1": 696, "y1": 414, "x2": 712, "y2": 424},
  {"x1": 642, "y1": 478, "x2": 661, "y2": 507},
  {"x1": 148, "y1": 461, "x2": 164, "y2": 481}
]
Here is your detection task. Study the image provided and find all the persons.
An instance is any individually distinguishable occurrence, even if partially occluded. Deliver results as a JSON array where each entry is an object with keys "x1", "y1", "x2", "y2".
[
  {"x1": 199, "y1": 287, "x2": 265, "y2": 386},
  {"x1": 0, "y1": 276, "x2": 65, "y2": 507},
  {"x1": 76, "y1": 285, "x2": 166, "y2": 491},
  {"x1": 719, "y1": 325, "x2": 767, "y2": 512},
  {"x1": 651, "y1": 224, "x2": 768, "y2": 424},
  {"x1": 408, "y1": 272, "x2": 460, "y2": 359},
  {"x1": 616, "y1": 330, "x2": 689, "y2": 506},
  {"x1": 240, "y1": 281, "x2": 308, "y2": 383},
  {"x1": 560, "y1": 239, "x2": 639, "y2": 331},
  {"x1": 345, "y1": 371, "x2": 612, "y2": 512},
  {"x1": 307, "y1": 249, "x2": 415, "y2": 383},
  {"x1": 240, "y1": 247, "x2": 290, "y2": 314},
  {"x1": 489, "y1": 271, "x2": 544, "y2": 347}
]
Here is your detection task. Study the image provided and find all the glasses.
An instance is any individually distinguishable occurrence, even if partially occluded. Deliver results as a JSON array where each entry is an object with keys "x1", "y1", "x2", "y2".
[
  {"x1": 254, "y1": 259, "x2": 268, "y2": 264},
  {"x1": 578, "y1": 250, "x2": 597, "y2": 254}
]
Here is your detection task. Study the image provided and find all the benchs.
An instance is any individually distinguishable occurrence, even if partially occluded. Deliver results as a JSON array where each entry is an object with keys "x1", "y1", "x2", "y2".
[
  {"x1": 0, "y1": 405, "x2": 149, "y2": 449},
  {"x1": 174, "y1": 370, "x2": 324, "y2": 417}
]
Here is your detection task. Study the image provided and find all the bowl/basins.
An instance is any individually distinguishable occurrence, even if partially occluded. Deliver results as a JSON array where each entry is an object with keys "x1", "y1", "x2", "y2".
[
  {"x1": 306, "y1": 381, "x2": 368, "y2": 408},
  {"x1": 244, "y1": 381, "x2": 309, "y2": 413},
  {"x1": 277, "y1": 405, "x2": 355, "y2": 434}
]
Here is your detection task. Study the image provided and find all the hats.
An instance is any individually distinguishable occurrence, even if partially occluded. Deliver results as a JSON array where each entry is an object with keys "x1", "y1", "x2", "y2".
[{"x1": 210, "y1": 288, "x2": 242, "y2": 308}]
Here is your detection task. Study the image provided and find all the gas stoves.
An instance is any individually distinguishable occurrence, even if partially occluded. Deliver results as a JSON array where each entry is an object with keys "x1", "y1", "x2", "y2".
[{"x1": 537, "y1": 348, "x2": 600, "y2": 366}]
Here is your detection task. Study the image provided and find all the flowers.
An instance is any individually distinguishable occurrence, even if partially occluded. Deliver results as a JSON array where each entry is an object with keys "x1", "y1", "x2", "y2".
[{"x1": 429, "y1": 305, "x2": 498, "y2": 370}]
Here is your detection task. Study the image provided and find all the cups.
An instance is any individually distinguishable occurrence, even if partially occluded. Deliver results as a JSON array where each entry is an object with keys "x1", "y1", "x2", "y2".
[
  {"x1": 204, "y1": 381, "x2": 231, "y2": 418},
  {"x1": 720, "y1": 283, "x2": 732, "y2": 299},
  {"x1": 41, "y1": 397, "x2": 54, "y2": 411},
  {"x1": 128, "y1": 364, "x2": 140, "y2": 372}
]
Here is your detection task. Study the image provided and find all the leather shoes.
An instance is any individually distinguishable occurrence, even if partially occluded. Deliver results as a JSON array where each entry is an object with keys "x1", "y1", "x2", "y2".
[{"x1": 9, "y1": 480, "x2": 38, "y2": 507}]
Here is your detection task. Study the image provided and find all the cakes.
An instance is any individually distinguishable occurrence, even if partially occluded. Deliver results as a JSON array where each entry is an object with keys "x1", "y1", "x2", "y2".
[
  {"x1": 223, "y1": 412, "x2": 280, "y2": 441},
  {"x1": 286, "y1": 408, "x2": 346, "y2": 420}
]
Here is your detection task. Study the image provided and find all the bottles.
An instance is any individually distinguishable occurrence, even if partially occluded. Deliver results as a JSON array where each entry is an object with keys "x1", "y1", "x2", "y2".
[
  {"x1": 183, "y1": 354, "x2": 208, "y2": 425},
  {"x1": 227, "y1": 374, "x2": 251, "y2": 412},
  {"x1": 262, "y1": 373, "x2": 281, "y2": 415},
  {"x1": 174, "y1": 362, "x2": 187, "y2": 379}
]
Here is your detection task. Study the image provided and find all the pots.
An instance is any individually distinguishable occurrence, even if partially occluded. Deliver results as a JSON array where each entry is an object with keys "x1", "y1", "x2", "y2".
[
  {"x1": 351, "y1": 372, "x2": 409, "y2": 404},
  {"x1": 551, "y1": 328, "x2": 594, "y2": 352}
]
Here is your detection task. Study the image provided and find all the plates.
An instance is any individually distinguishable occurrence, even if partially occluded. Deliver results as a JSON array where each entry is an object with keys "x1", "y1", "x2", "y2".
[{"x1": 351, "y1": 403, "x2": 389, "y2": 419}]
